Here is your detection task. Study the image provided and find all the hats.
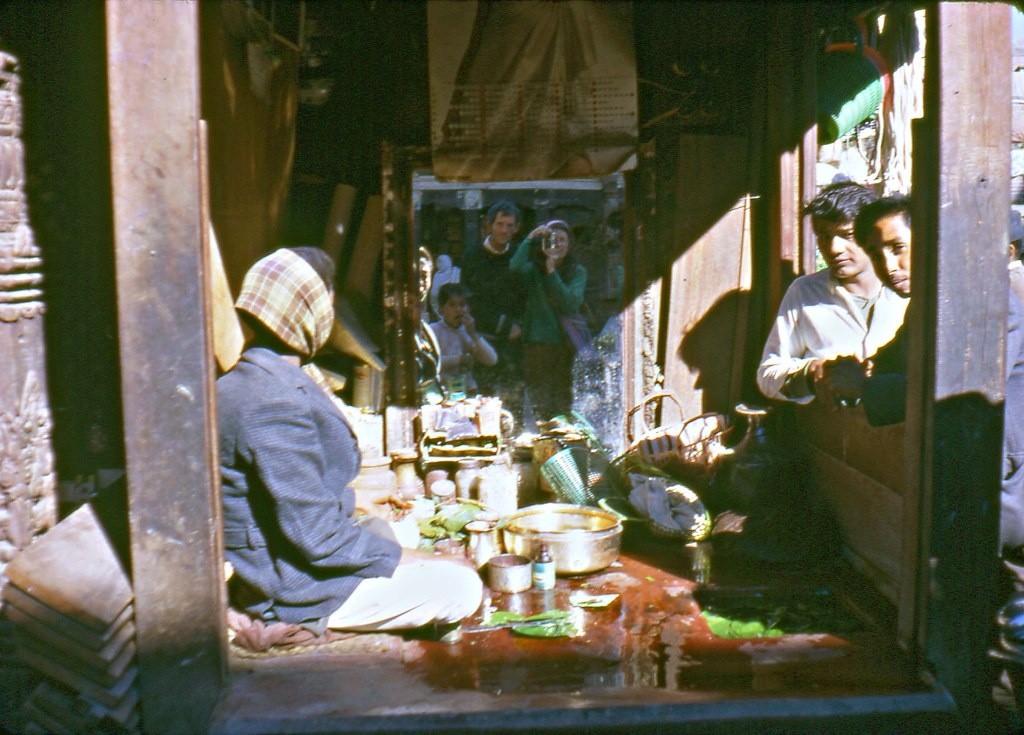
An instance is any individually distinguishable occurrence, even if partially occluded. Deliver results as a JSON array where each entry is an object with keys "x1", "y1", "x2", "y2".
[{"x1": 547, "y1": 220, "x2": 569, "y2": 234}]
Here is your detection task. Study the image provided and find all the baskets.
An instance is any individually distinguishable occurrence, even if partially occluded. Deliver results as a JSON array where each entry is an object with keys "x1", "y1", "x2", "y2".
[
  {"x1": 532, "y1": 434, "x2": 590, "y2": 493},
  {"x1": 610, "y1": 393, "x2": 738, "y2": 491},
  {"x1": 629, "y1": 477, "x2": 711, "y2": 541},
  {"x1": 539, "y1": 445, "x2": 627, "y2": 511}
]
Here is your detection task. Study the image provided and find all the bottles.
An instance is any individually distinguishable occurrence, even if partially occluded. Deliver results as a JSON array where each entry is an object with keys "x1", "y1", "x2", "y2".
[
  {"x1": 454, "y1": 460, "x2": 482, "y2": 501},
  {"x1": 533, "y1": 544, "x2": 555, "y2": 589},
  {"x1": 477, "y1": 459, "x2": 517, "y2": 515},
  {"x1": 391, "y1": 452, "x2": 425, "y2": 499},
  {"x1": 350, "y1": 458, "x2": 396, "y2": 509}
]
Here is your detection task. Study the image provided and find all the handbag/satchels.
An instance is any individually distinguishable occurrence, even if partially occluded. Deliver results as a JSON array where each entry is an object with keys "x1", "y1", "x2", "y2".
[{"x1": 558, "y1": 312, "x2": 597, "y2": 357}]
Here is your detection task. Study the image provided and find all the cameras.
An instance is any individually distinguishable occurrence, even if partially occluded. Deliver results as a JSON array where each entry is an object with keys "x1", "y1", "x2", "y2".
[{"x1": 541, "y1": 231, "x2": 556, "y2": 251}]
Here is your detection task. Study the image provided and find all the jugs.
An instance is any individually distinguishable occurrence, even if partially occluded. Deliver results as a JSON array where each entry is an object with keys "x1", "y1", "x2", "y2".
[{"x1": 466, "y1": 521, "x2": 499, "y2": 576}]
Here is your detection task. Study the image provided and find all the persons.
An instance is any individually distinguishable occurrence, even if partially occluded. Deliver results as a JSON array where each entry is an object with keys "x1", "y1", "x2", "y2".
[
  {"x1": 214, "y1": 248, "x2": 484, "y2": 639},
  {"x1": 420, "y1": 203, "x2": 588, "y2": 421},
  {"x1": 757, "y1": 182, "x2": 1024, "y2": 560}
]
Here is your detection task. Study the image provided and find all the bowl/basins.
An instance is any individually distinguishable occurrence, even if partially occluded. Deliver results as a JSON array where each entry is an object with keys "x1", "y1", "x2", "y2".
[
  {"x1": 504, "y1": 509, "x2": 623, "y2": 574},
  {"x1": 487, "y1": 555, "x2": 532, "y2": 594}
]
